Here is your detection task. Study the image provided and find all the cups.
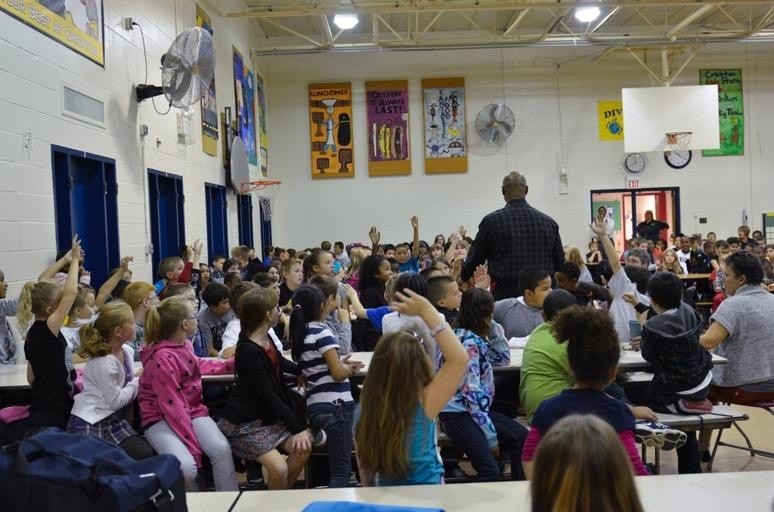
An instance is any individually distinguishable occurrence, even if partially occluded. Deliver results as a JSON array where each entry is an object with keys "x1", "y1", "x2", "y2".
[{"x1": 629, "y1": 321, "x2": 641, "y2": 351}]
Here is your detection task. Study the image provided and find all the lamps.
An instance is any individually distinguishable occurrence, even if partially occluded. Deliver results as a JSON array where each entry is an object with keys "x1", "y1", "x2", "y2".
[
  {"x1": 333, "y1": 12, "x2": 359, "y2": 30},
  {"x1": 573, "y1": 7, "x2": 601, "y2": 22}
]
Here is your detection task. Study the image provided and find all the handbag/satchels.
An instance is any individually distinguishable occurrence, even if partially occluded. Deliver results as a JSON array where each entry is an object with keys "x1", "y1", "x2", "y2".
[{"x1": 0, "y1": 428, "x2": 188, "y2": 512}]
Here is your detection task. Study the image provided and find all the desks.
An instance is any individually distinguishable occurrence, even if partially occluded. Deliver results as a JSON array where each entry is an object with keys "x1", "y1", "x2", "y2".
[
  {"x1": 0, "y1": 344, "x2": 728, "y2": 393},
  {"x1": 676, "y1": 273, "x2": 711, "y2": 280},
  {"x1": 186, "y1": 490, "x2": 240, "y2": 511},
  {"x1": 230, "y1": 471, "x2": 774, "y2": 511}
]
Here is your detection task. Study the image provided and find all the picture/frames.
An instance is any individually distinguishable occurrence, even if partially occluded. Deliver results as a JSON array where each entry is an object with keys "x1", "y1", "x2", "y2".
[{"x1": 0, "y1": 0, "x2": 105, "y2": 69}]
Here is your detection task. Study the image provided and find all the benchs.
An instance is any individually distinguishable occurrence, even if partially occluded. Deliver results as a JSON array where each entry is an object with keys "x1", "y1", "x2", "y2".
[
  {"x1": 439, "y1": 404, "x2": 750, "y2": 473},
  {"x1": 695, "y1": 301, "x2": 713, "y2": 326}
]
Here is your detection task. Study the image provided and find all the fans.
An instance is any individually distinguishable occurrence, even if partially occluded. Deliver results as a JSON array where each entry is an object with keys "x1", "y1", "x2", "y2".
[
  {"x1": 136, "y1": 27, "x2": 219, "y2": 109},
  {"x1": 475, "y1": 104, "x2": 516, "y2": 144}
]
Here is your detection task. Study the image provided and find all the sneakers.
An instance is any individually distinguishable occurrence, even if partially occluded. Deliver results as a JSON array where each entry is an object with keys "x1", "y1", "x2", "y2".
[
  {"x1": 674, "y1": 398, "x2": 713, "y2": 415},
  {"x1": 444, "y1": 467, "x2": 472, "y2": 483},
  {"x1": 698, "y1": 450, "x2": 709, "y2": 461},
  {"x1": 634, "y1": 417, "x2": 687, "y2": 452}
]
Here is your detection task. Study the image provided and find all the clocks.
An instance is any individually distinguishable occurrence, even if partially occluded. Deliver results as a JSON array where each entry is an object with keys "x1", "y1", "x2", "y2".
[
  {"x1": 625, "y1": 153, "x2": 645, "y2": 174},
  {"x1": 664, "y1": 150, "x2": 691, "y2": 169}
]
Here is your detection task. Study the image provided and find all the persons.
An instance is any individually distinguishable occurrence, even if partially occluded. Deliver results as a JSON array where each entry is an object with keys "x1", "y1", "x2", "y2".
[
  {"x1": 592, "y1": 206, "x2": 615, "y2": 244},
  {"x1": 638, "y1": 211, "x2": 669, "y2": 242},
  {"x1": 458, "y1": 172, "x2": 565, "y2": 302},
  {"x1": 0, "y1": 215, "x2": 773, "y2": 511}
]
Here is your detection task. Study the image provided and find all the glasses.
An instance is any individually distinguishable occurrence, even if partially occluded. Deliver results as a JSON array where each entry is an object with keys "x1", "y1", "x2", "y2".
[
  {"x1": 185, "y1": 313, "x2": 200, "y2": 321},
  {"x1": 138, "y1": 296, "x2": 159, "y2": 305}
]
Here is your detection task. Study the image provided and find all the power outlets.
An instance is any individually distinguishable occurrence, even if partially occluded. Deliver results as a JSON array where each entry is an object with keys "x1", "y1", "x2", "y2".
[{"x1": 125, "y1": 17, "x2": 134, "y2": 30}]
